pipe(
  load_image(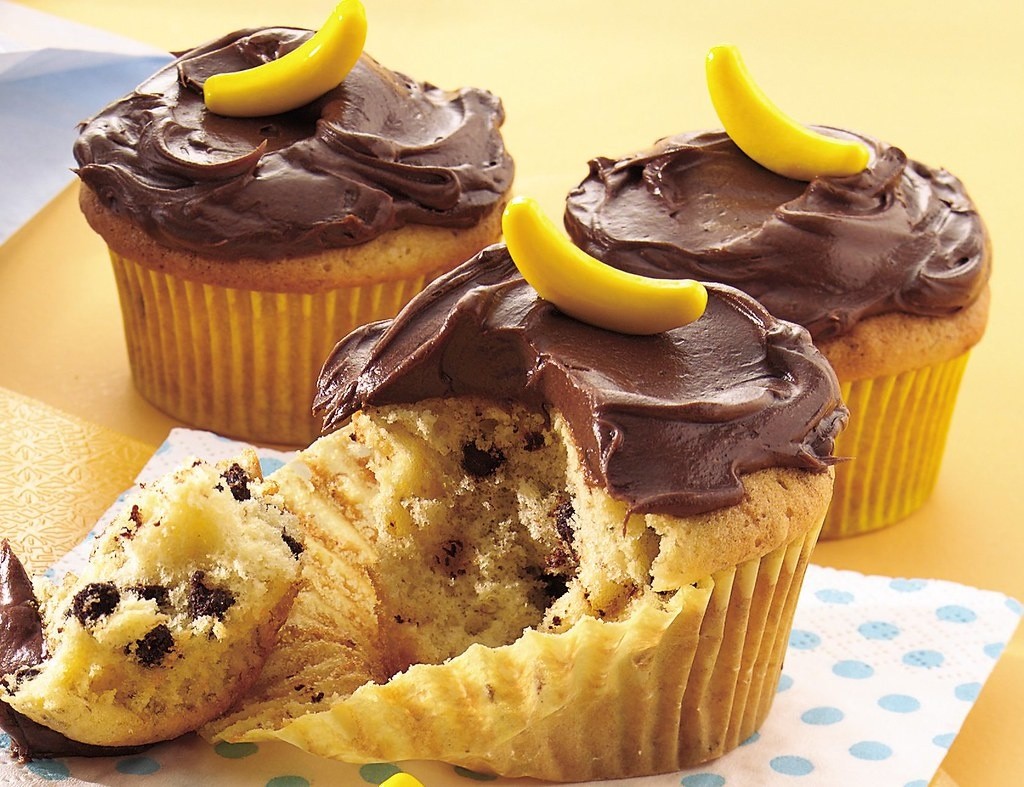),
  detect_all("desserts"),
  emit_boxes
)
[0,1,994,787]
